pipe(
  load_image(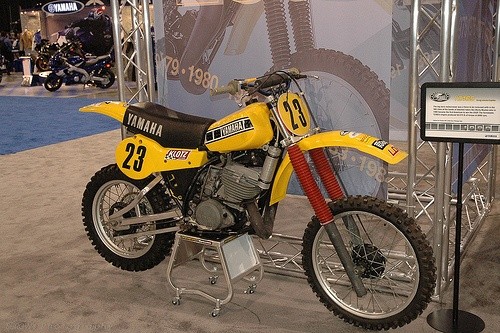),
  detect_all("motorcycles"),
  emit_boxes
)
[44,43,115,92]
[34,39,71,71]
[78,68,438,333]
